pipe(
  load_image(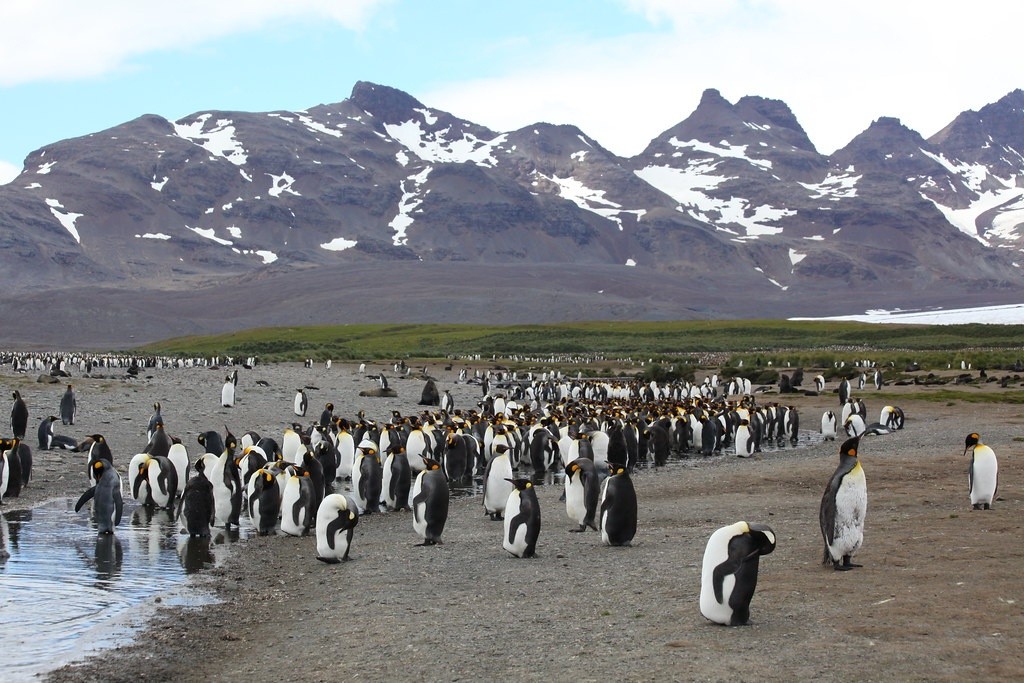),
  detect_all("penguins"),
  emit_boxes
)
[963,433,998,511]
[559,457,600,532]
[316,493,359,564]
[819,430,867,570]
[598,459,638,549]
[502,478,541,559]
[413,454,449,546]
[699,520,776,626]
[0,340,1023,536]
